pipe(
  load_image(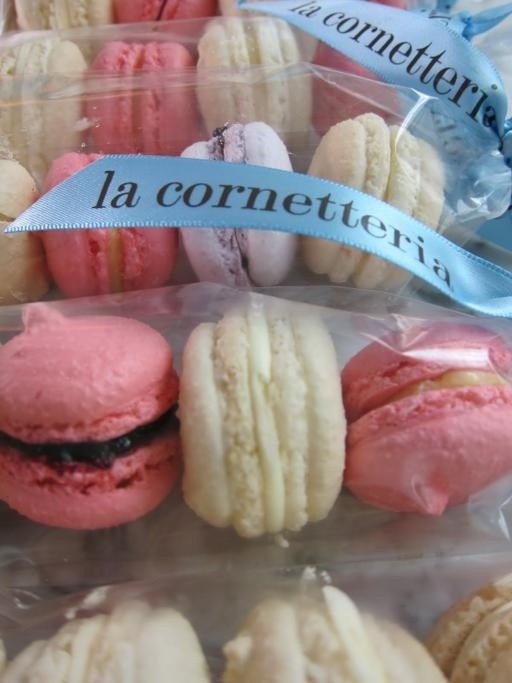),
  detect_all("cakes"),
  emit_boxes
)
[0,0,512,683]
